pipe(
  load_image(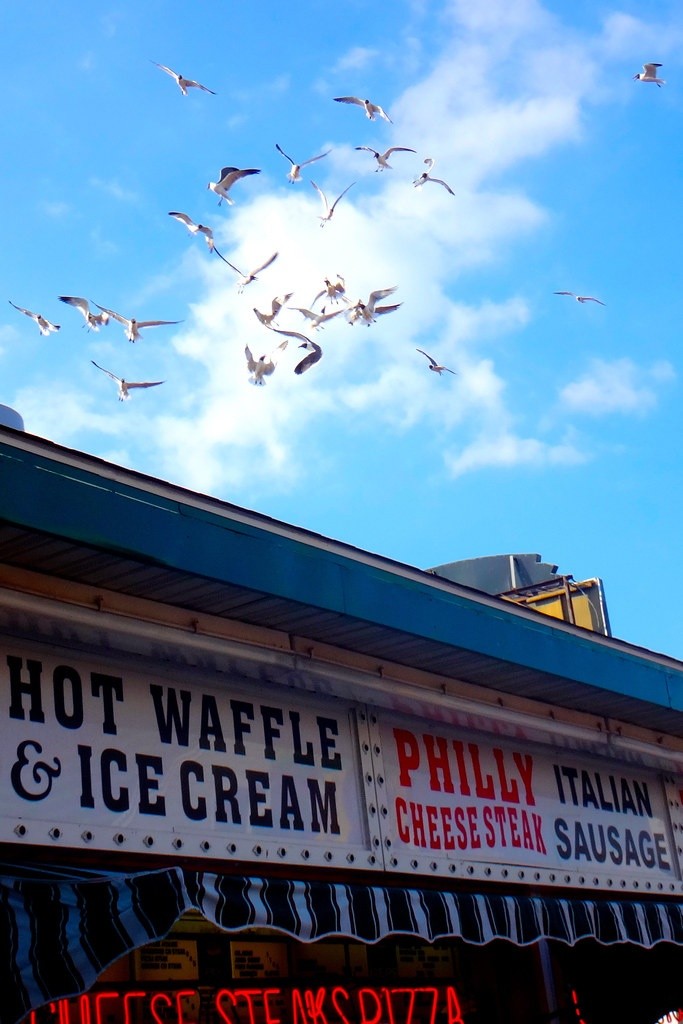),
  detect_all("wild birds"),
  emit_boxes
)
[415,347,458,376]
[149,59,221,98]
[91,359,166,402]
[163,163,279,293]
[551,291,608,307]
[633,61,670,90]
[243,270,406,386]
[275,94,455,229]
[8,296,187,344]
